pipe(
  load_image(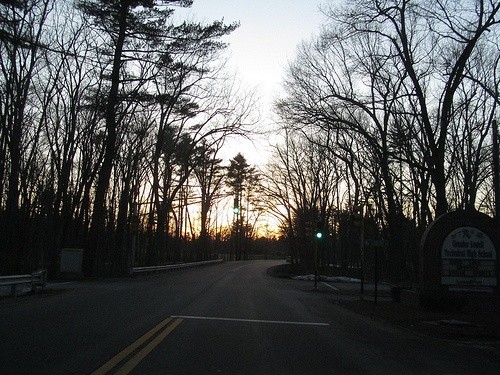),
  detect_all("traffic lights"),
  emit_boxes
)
[315,221,324,240]
[234,198,239,214]
